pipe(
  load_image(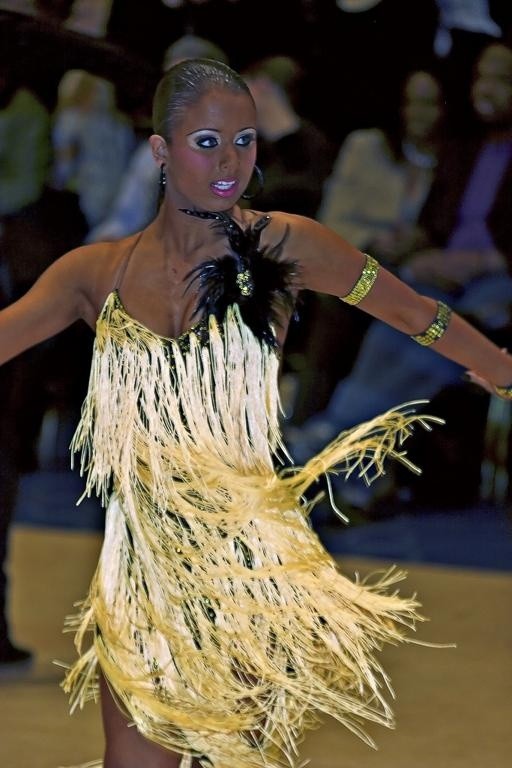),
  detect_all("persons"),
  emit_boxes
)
[0,61,512,768]
[1,1,511,526]
[0,93,90,665]
